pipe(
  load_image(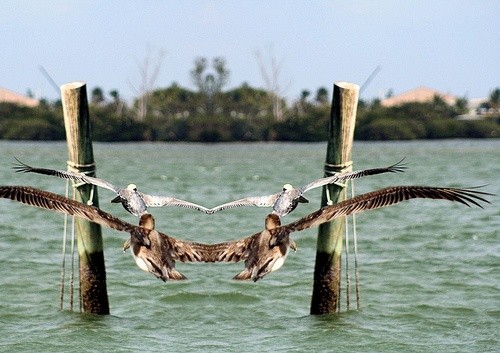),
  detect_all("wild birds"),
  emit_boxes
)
[210,182,498,283]
[209,156,409,217]
[0,186,210,283]
[10,157,209,217]
